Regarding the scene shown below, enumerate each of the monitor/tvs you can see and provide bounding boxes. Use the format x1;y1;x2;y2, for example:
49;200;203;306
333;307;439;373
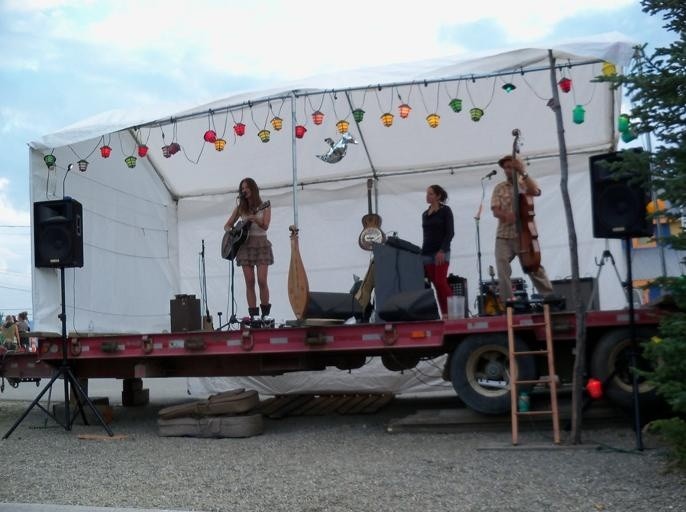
33;198;84;268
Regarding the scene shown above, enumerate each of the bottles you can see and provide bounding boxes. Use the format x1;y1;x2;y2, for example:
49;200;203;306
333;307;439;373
88;320;95;337
518;390;530;414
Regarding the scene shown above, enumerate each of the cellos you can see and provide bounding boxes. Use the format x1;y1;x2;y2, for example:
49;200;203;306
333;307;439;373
512;129;541;275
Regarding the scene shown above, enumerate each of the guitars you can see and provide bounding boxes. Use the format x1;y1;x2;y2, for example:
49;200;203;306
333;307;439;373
359;179;386;251
221;200;270;261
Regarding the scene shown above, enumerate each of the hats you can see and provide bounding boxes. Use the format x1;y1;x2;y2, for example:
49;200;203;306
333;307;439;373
498;154;521;164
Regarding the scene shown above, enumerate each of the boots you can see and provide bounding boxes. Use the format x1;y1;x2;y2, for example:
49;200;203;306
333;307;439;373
260;304;274;322
248;308;261;323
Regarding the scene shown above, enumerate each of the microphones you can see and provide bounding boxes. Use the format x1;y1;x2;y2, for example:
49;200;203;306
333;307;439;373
200;239;205;258
486;169;497;178
237;191;246;199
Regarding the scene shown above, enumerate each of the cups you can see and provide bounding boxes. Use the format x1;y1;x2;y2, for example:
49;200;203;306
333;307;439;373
446;296;465;320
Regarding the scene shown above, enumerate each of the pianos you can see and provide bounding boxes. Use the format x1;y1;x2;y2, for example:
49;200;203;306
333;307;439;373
387;237;422;254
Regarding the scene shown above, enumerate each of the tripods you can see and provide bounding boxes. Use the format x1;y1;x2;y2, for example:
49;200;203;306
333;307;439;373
615;240;672;454
213;199;245;331
2;269;114;440
585;239;628;310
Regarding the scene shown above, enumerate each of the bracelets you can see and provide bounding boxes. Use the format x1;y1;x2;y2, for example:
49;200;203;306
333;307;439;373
522;173;528;180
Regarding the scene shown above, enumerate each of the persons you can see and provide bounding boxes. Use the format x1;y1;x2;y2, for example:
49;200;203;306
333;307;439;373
422;184;455;321
491;156;556;303
224;177;274;320
0;312;31;351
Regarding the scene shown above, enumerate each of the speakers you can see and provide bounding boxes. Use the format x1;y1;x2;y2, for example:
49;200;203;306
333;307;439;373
170;298;201;332
588;148;655;240
302;291;372;324
376;289;441;320
